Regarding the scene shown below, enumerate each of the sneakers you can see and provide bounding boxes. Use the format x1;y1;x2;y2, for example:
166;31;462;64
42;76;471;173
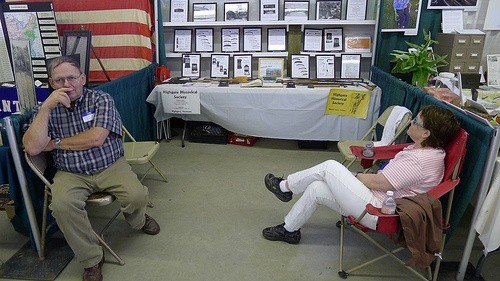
83;248;104;281
263;222;302;244
142;213;160;235
265;173;293;202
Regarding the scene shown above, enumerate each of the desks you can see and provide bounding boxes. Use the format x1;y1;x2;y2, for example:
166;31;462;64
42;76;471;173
146;76;382;148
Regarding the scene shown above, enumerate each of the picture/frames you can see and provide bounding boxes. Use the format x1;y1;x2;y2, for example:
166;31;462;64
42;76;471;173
426;0;479;10
381;0;423;32
62;29;93;86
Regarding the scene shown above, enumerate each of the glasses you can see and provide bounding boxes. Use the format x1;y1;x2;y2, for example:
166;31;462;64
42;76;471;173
413;117;423;127
52;73;82;85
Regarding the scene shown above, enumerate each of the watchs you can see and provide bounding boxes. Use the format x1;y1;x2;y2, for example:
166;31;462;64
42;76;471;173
352;171;362;177
55;137;63;150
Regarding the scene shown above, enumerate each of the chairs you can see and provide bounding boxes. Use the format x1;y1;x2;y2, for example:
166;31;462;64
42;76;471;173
121;122;167;208
337;126;469;281
25;144;125;266
338;105;412;174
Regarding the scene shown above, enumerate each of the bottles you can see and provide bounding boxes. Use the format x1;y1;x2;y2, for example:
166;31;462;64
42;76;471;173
362;141;375;158
380;190;396;215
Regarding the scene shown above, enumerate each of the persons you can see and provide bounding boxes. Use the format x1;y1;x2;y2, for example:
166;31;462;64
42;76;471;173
22;56;160;281
263;105;461;244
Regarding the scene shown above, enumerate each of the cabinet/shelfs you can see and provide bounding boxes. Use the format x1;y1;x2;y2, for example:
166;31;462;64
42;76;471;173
434;31;486;74
162;17;376;58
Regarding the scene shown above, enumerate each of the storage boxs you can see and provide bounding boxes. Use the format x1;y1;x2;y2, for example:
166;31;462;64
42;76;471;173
187;120;228;144
